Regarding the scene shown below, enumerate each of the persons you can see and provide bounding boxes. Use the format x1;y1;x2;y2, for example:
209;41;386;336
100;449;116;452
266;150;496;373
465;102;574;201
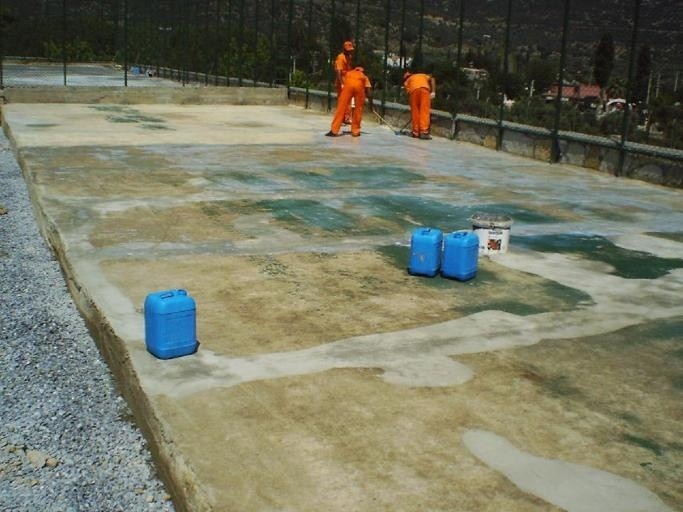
334;40;354;126
324;66;374;137
402;70;435;139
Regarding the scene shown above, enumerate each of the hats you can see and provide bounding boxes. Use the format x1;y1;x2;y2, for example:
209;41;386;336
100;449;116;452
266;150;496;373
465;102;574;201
343;40;355;52
403;73;412;79
355;66;364;71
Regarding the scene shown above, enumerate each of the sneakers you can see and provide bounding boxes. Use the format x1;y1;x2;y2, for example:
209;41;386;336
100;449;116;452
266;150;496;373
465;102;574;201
419;133;433;139
325;130;336;136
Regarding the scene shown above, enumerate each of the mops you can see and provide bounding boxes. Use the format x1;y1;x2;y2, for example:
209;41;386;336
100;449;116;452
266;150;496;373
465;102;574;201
372;109;413;136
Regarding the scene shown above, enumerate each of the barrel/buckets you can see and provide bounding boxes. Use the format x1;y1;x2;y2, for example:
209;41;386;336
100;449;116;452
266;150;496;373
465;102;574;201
407;227;444;278
441;231;480;281
467;211;514;256
142;289;200;360
132;66;140;75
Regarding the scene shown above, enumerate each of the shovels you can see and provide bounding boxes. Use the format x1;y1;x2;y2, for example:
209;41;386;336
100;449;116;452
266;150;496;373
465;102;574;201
373;111;405;136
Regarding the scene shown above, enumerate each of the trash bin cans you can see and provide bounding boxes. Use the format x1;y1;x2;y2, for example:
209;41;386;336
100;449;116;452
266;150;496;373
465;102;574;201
471;213;514;253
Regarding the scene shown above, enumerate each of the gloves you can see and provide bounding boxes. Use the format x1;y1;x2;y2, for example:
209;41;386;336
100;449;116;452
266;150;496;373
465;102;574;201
430;92;435;99
